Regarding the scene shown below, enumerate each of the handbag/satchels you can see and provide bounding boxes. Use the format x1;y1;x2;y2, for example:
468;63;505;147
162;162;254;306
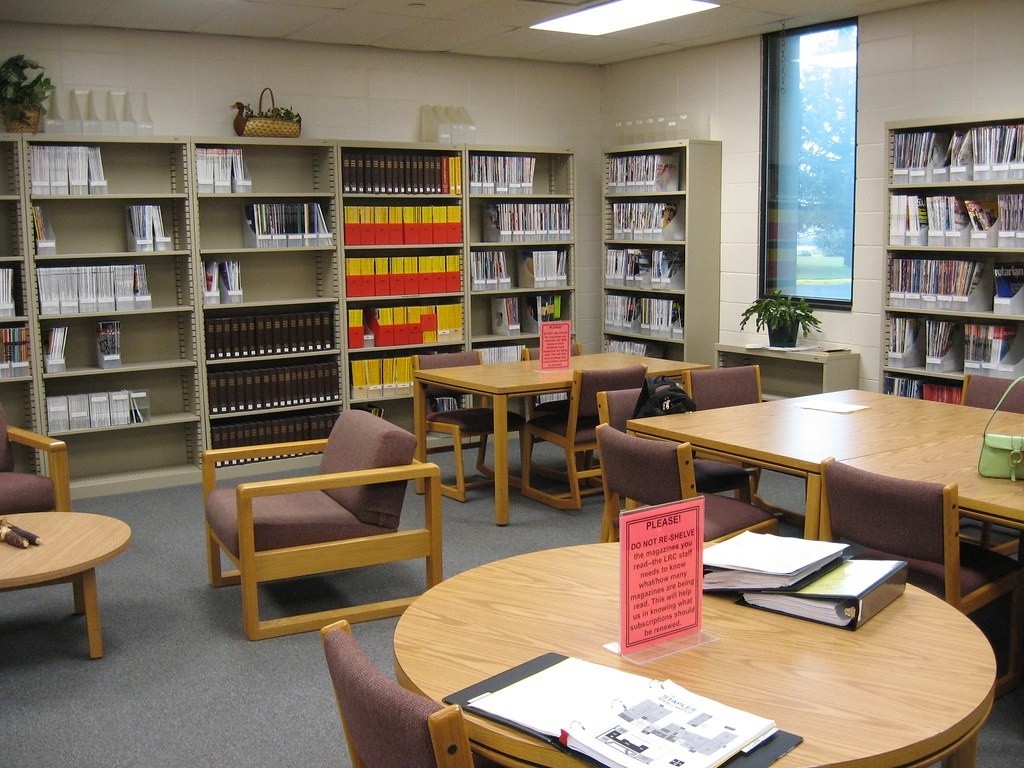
977;376;1024;481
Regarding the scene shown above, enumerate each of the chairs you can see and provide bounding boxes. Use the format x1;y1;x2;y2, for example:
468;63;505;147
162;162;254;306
207;409;445;640
522;342;584;359
413;352;527;501
321;620;473;767
959;374;1024;415
681;366;765;409
1;426;76;513
596;423;779;547
596;385;755;504
818;456;1024;700
519;367;645;510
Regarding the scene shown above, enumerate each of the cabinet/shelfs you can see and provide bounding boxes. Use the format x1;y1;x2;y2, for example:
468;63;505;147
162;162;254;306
192;133;344;467
338;139;468;449
880;116;1024;408
22;134;205;500
1;132;45;480
596;139;721;368
713;343;860;402
466;145;575;362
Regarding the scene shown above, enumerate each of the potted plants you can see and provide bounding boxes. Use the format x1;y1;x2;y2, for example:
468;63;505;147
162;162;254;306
742;292;819;349
1;55;53;134
231;88;301;138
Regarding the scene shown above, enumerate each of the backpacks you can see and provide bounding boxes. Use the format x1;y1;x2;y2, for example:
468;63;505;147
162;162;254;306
633;375;696;459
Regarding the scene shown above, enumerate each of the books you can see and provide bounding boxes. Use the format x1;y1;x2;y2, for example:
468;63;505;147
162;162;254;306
605;153;682;358
0;269;15;316
887;123;1024;407
201;260;242;305
245;202;332;247
469;156;570;404
47;387;144;432
32;205;47;255
36;263;149;315
195;146;245;193
0;327;29;377
341;149;463;418
128;204;166;251
31;146;106;195
42;325;68;373
204;312;341;466
96;321;121;367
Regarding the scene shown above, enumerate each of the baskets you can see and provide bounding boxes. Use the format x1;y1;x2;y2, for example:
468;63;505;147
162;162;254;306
2;103;40;133
240;87;301;137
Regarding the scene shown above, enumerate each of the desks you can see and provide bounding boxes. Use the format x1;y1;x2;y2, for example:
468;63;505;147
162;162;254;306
410;353;712;526
393;539;996;768
627;389;1024;545
1;507;130;661
